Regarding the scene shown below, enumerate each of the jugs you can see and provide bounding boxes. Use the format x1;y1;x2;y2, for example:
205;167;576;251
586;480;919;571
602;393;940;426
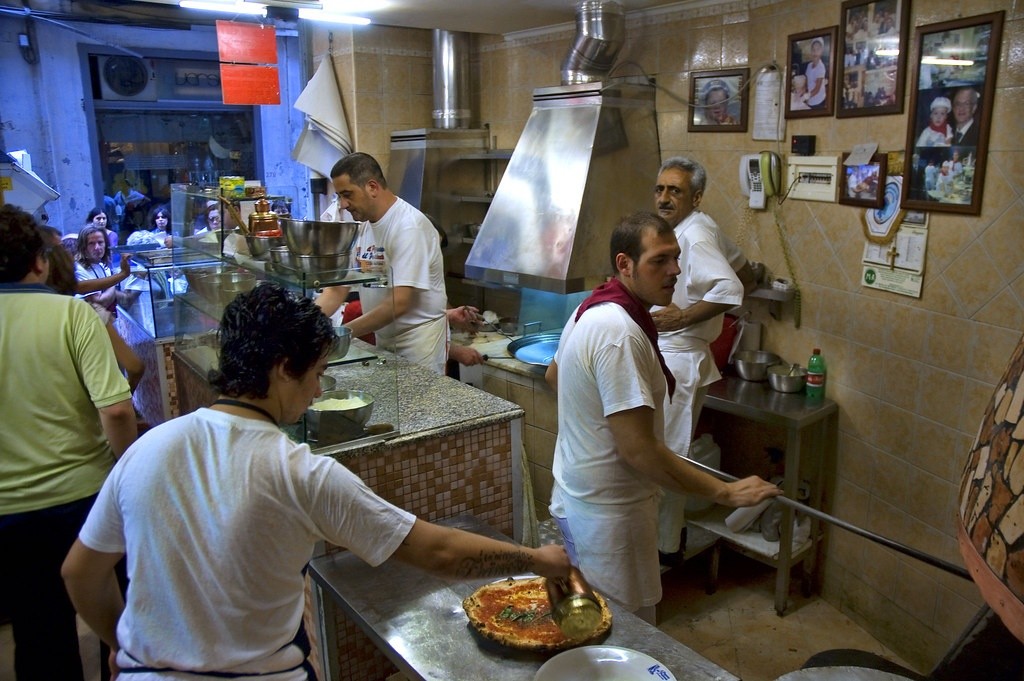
217;195;282;236
545;565;602;637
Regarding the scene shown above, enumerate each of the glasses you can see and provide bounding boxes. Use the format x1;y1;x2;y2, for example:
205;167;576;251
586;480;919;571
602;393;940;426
207;216;222;222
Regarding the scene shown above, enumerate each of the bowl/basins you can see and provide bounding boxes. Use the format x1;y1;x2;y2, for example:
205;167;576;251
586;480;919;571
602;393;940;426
304;374;375;438
183;266;279;310
496;315;517;330
248;217;361;281
733;351;782;381
468;223;482;238
475;319;497;332
766;365;808;393
330;325;352;361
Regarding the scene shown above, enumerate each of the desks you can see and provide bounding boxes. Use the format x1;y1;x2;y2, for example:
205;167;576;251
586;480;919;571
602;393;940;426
686;372;837;617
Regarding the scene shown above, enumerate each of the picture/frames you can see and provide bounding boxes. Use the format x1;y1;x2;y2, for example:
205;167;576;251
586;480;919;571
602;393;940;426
836;0;911;120
686;67;749;133
784;24;839;120
899;10;1007;216
839;151;888;210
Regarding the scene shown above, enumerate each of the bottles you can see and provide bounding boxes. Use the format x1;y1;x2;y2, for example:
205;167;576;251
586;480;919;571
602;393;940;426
806;347;825;400
682;435;723;519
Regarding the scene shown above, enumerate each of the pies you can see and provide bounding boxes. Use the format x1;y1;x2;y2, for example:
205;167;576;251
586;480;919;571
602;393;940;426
462;575;613;650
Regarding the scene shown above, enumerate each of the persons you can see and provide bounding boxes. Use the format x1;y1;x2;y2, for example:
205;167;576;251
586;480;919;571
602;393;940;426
794;37;826;109
164;199;252;273
115;230;164;334
951;88;980;146
39;207;144;394
916;98;953;146
650;157;757;554
699;79;736;125
313;152;451;376
151;208;172;236
104;179;150;231
0;201;136;681
919;34;962;90
271;200;291;214
546;212;783;626
340;300;485;367
61;281;571;681
908;150;962;202
847;167;877;198
843;9;896;107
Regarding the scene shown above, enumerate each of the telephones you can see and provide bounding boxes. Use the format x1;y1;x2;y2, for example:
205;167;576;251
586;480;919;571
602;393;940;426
739;154;767;209
761;151;781;196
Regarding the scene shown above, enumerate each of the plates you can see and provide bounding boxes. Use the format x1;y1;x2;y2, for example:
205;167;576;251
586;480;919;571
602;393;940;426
293;420;366;442
533;645;677;681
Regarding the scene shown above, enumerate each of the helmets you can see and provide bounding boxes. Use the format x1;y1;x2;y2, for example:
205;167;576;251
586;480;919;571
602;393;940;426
930;97;952;113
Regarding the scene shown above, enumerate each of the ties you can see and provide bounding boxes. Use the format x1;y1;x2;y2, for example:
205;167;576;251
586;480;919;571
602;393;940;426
929;119;947;137
955;131;963;142
574;277;675;403
